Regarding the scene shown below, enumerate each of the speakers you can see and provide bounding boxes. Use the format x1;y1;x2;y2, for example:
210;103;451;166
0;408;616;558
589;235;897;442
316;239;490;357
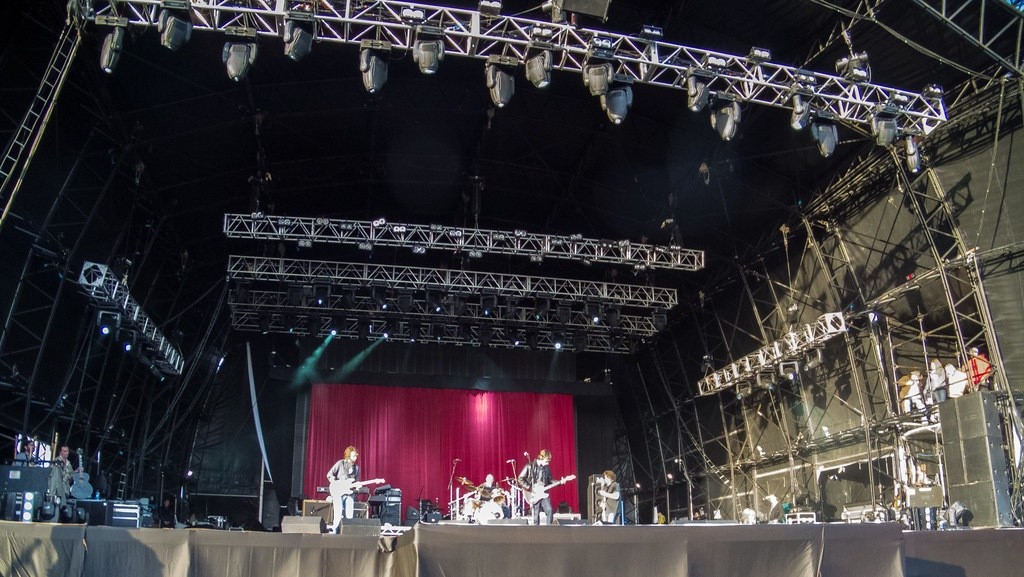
487;519;528;524
587;483;603;518
551;518;591;525
281;499;443;536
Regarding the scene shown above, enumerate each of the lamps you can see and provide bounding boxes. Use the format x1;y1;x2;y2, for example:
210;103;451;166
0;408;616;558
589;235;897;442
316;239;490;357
222;26;259;83
522;42;554;89
600;74;635;124
710;92;743;141
681;67;713;112
284;10;316;63
703;56;726;71
485;55;520;109
583;51;613;96
528;26;553;40
589;36;613;50
156;1;193;50
412;24;446;76
400;6;425;24
749;46;943;179
94;215;823;403
478;0;504;15
95;14;128;72
639;25;664;40
358;37;391;94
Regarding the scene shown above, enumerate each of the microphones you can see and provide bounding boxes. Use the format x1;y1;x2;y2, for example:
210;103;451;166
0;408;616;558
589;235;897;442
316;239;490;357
454;459;460;461
311;509;313;516
524;452;529;456
506;459;515;463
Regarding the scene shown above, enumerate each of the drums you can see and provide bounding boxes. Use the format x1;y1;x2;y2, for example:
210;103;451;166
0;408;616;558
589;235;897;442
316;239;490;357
491;488;505;504
463;498;481;516
474;502;504;524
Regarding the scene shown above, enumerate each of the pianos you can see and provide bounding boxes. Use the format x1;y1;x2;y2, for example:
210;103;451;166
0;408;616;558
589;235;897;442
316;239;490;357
316;486;370;525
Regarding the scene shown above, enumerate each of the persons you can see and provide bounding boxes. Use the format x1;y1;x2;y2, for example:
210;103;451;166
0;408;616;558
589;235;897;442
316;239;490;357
923;358;946;423
54;445;84;495
944;363;967;399
598;470;620;524
960;347;993;393
694;509;704;520
896;369;928;425
475;474;499;499
16;440;36;467
326;446;362;534
517;449;566;525
763;494;783;522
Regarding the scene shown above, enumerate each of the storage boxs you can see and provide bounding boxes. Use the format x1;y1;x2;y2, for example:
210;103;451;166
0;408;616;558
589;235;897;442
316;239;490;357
112;504;143;528
786;512;816;525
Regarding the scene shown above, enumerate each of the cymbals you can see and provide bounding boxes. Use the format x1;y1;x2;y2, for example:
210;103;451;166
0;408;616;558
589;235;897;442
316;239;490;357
456;477;473;484
466;486;488;495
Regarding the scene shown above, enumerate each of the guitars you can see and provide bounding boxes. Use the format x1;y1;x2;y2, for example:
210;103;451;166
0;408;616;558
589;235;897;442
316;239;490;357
69;448;94;499
329;478;385;499
523;474;577;505
601;483;606;512
